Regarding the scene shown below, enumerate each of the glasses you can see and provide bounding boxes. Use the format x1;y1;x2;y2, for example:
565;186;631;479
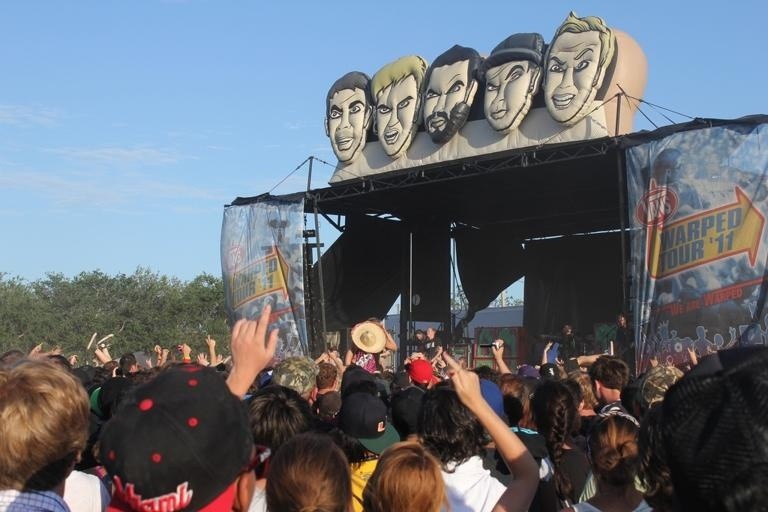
237;445;271;479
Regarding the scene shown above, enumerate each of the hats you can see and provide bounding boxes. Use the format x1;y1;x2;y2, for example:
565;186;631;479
479;379;504;417
88;371;256;512
273;356;320;393
539;363;560;382
351;321;386;354
342;360;438;455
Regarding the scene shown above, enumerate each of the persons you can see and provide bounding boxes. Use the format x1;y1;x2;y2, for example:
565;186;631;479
484;32;545;134
542;9;617;126
423;44;483;147
323;69;373;165
632;127;768;338
221;202;308;360
367;55;428;159
